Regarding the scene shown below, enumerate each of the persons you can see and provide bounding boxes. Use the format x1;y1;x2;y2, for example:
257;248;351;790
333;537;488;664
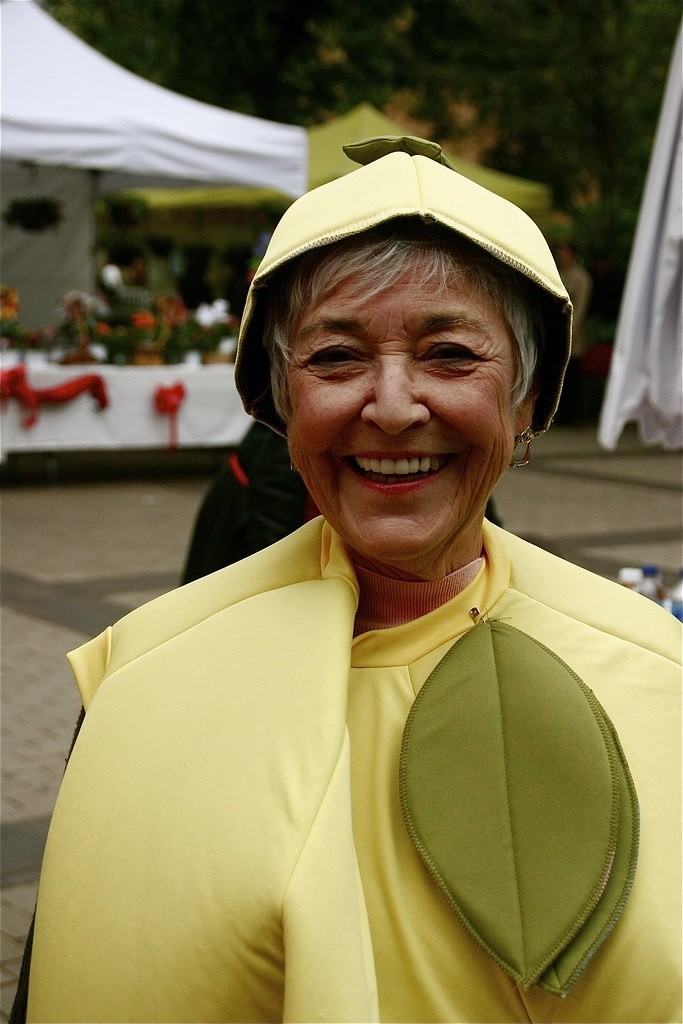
11;136;683;1022
0;285;95;352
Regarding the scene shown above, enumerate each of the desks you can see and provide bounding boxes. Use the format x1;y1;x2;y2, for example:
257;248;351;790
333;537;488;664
2;343;254;483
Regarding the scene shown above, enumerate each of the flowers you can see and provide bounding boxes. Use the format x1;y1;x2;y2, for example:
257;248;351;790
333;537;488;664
0;269;238;366
154;384;184;414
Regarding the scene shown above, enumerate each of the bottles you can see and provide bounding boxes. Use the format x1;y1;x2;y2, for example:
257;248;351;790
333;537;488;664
640;567;667;607
668;566;683;624
617;566;644;591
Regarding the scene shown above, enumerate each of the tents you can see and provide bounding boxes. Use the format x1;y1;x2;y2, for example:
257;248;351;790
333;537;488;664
2;0;548;233
